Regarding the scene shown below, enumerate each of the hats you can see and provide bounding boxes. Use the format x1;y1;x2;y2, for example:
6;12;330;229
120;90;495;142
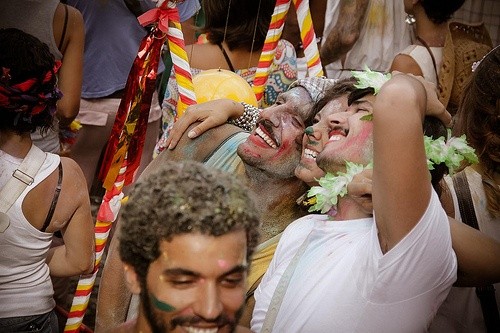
437;20;495;110
288;78;340;103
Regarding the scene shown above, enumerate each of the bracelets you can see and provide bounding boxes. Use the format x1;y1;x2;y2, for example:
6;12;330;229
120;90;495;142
229;101;263;132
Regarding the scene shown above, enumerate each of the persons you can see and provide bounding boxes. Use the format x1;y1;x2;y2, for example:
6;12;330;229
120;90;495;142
0;0;500;333
